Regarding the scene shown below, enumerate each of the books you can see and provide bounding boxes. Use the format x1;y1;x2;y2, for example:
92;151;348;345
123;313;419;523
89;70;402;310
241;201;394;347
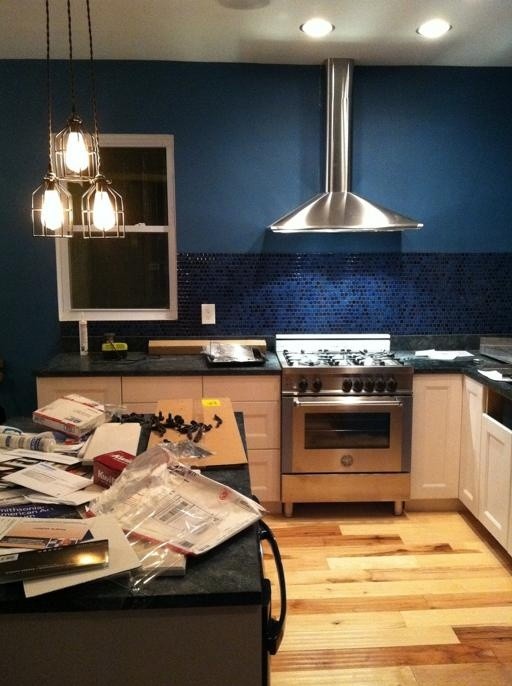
81;421;143;466
33;397;106;439
104;540;187;579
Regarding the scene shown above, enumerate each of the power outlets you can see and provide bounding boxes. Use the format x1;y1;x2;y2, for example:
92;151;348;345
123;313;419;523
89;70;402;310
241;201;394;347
202;304;216;324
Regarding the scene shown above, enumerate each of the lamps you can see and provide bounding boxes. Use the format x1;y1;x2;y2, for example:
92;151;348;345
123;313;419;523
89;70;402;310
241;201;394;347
32;0;75;238
81;0;126;240
54;0;98;182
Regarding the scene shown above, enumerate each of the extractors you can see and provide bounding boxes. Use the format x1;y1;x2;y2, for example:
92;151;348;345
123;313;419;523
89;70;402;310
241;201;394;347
266;58;424;234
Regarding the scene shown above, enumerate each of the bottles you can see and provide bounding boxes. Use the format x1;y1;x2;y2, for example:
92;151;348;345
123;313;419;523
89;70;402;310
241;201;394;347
0;434;71;453
79;312;88;356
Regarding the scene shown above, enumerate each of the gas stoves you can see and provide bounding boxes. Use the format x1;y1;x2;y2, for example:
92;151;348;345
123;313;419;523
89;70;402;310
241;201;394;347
277;349;414;395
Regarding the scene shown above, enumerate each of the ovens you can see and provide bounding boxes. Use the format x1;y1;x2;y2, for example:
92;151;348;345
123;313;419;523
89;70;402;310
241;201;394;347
281;395;413;517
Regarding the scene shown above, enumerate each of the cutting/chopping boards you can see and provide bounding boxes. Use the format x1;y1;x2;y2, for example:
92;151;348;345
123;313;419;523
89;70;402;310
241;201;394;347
81;469;201;577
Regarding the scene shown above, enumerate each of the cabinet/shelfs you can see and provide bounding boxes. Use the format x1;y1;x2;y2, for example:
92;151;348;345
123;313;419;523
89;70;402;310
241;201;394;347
411;368;463;512
458;369;487;531
37;370;122;421
122;370;203;414
203;369;282;514
50;134;179;322
479;377;512;557
